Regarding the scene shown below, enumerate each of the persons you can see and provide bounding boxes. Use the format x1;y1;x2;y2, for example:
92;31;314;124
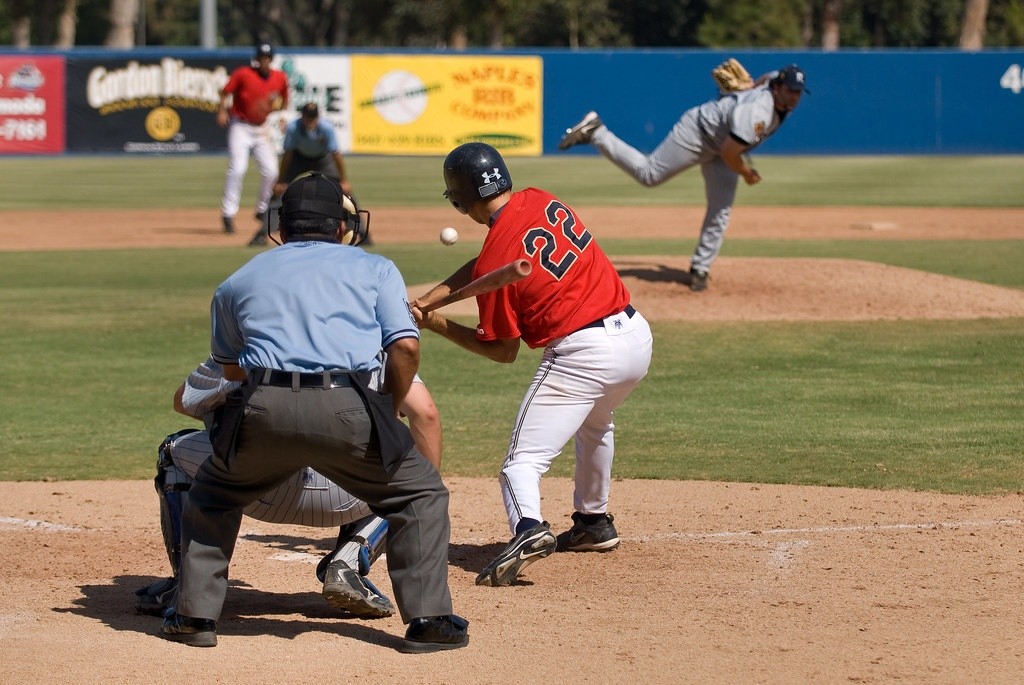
248;102;373;245
140;171;469;653
410;142;653;583
216;43;288;234
558;58;810;292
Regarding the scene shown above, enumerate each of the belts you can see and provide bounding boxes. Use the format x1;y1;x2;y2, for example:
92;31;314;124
568;303;637;335
698;110;707;136
258;369;354;388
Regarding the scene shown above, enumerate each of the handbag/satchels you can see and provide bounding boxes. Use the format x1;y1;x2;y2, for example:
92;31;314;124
351;371;415;474
210;366;265;465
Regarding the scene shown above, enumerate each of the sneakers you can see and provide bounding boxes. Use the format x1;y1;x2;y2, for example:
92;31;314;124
560;110;603;149
400;614;470;653
690;268;711;292
158;606;218;646
555;512;621;554
475;520;557;588
135;576;177;618
322;560;396;619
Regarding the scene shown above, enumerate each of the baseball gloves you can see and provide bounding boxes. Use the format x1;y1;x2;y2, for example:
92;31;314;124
712;57;755;93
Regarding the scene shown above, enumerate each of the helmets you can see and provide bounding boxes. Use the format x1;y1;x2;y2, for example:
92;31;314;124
443;142;512;215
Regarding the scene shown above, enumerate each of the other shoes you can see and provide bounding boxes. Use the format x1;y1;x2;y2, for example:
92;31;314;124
256;211;266;221
222;215;236;233
249;230;266;247
359;230;373;245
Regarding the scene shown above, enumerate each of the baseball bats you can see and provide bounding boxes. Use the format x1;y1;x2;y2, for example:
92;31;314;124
419;259;532;312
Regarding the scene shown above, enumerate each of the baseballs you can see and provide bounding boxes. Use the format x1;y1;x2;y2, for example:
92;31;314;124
439;227;459;246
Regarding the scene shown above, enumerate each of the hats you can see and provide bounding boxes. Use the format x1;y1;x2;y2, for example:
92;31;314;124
301;104;318;118
281;172;343;220
256;44;272;63
779;66;811;95
